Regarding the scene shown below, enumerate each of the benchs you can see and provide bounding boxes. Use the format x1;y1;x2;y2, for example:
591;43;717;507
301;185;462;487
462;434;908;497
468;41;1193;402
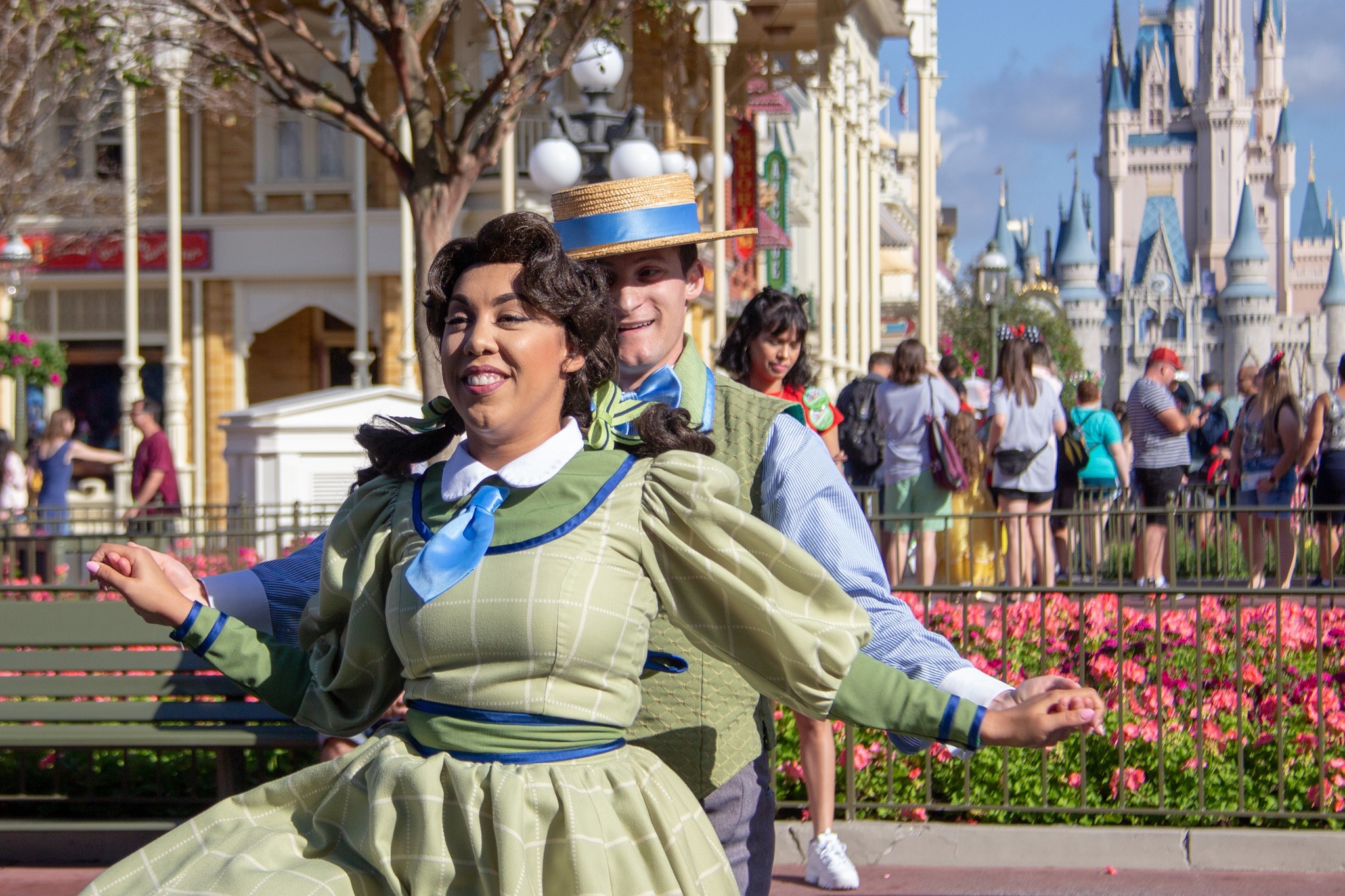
0;601;332;832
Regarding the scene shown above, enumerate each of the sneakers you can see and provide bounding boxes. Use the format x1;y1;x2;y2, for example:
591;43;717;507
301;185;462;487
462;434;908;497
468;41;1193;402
804;828;859;890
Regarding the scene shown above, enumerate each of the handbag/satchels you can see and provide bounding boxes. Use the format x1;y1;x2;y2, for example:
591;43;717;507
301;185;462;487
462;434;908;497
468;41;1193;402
1199;429;1235;497
997;449;1037;474
923;377;967;494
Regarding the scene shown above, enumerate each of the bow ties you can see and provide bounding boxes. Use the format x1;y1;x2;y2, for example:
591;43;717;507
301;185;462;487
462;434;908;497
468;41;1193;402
590;363;682;410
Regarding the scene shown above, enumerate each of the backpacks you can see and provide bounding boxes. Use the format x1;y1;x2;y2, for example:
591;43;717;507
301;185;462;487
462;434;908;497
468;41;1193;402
1073;408;1103;453
1193;397;1231;456
838;377;884;471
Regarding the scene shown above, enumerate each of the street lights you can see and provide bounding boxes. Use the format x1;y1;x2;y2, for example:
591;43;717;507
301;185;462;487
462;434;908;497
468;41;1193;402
93;3;198;560
974;242;1011;382
0;232;36;463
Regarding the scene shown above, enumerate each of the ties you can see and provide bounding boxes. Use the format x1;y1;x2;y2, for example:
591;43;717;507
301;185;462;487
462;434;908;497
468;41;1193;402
404;486;512;602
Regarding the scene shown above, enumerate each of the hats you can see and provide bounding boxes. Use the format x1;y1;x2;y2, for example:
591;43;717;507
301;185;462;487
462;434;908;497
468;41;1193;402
1201;371;1225;386
550;172;757;262
1146;348;1182;371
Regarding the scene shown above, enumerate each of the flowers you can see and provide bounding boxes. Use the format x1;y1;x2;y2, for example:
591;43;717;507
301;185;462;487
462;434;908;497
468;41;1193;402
0;331;69;389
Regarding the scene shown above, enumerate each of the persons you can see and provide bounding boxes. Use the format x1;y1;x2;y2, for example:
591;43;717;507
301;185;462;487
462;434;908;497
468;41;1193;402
78;213;1106;896
835;351;894;531
122;399;182;556
875;339;961;611
108;172;1107;896
938;354;966;401
1182;371;1242;540
934;410;1006;604
1125;348;1210;600
717;287;859;889
1068;381;1133;571
25;408;127;537
1299;354;1345;588
984;337;1077;604
0;430;29;535
1229;351;1302;591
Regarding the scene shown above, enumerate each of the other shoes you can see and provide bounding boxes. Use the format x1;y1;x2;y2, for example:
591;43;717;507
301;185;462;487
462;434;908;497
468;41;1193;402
1055;573;1068;584
1310;571;1335;586
1139;576;1184;600
